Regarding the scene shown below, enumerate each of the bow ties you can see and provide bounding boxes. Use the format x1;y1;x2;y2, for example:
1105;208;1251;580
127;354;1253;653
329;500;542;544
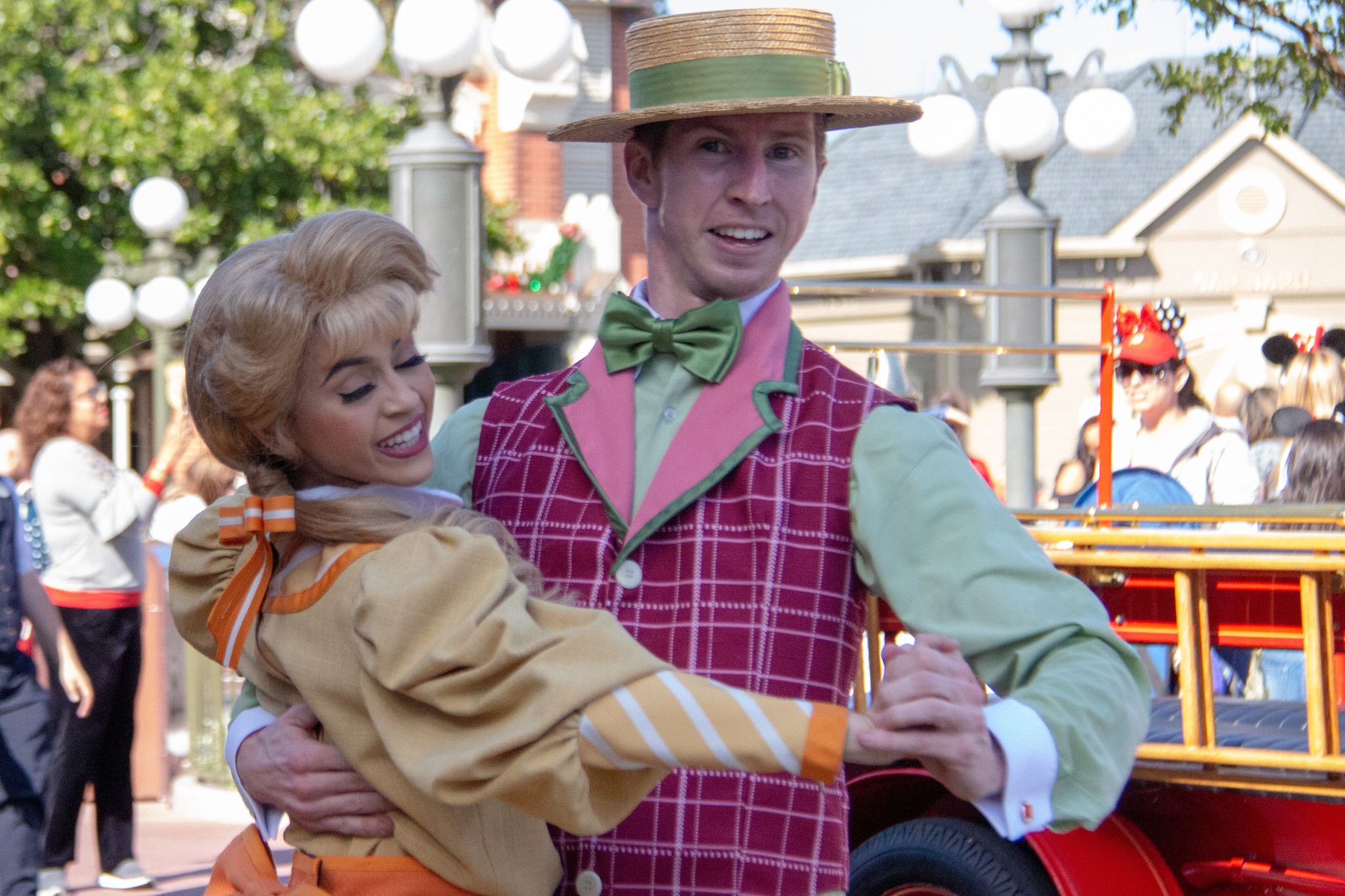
596;289;742;384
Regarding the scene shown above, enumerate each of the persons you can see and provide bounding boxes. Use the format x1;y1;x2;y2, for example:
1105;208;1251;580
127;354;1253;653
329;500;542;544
1093;299;1259;700
1054;414;1099;528
1216;329;1345;499
14;356;192;896
1258;419;1345;703
225;8;1151;896
170;210;953;896
903;393;992;492
0;472;93;896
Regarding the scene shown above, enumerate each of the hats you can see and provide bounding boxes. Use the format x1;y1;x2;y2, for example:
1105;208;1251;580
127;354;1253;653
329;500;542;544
544;7;922;143
1113;298;1184;366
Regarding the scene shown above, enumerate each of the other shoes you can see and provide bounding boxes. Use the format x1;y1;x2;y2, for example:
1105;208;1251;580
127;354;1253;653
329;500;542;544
36;867;68;896
97;860;154;889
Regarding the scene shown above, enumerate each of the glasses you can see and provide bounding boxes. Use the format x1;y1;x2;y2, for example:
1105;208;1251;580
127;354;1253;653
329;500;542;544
73;382;107;404
1115;362;1171;383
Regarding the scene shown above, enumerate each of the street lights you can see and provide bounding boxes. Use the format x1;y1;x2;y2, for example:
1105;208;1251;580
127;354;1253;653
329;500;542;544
910;8;1139;527
295;0;574;442
82;175;210;720
